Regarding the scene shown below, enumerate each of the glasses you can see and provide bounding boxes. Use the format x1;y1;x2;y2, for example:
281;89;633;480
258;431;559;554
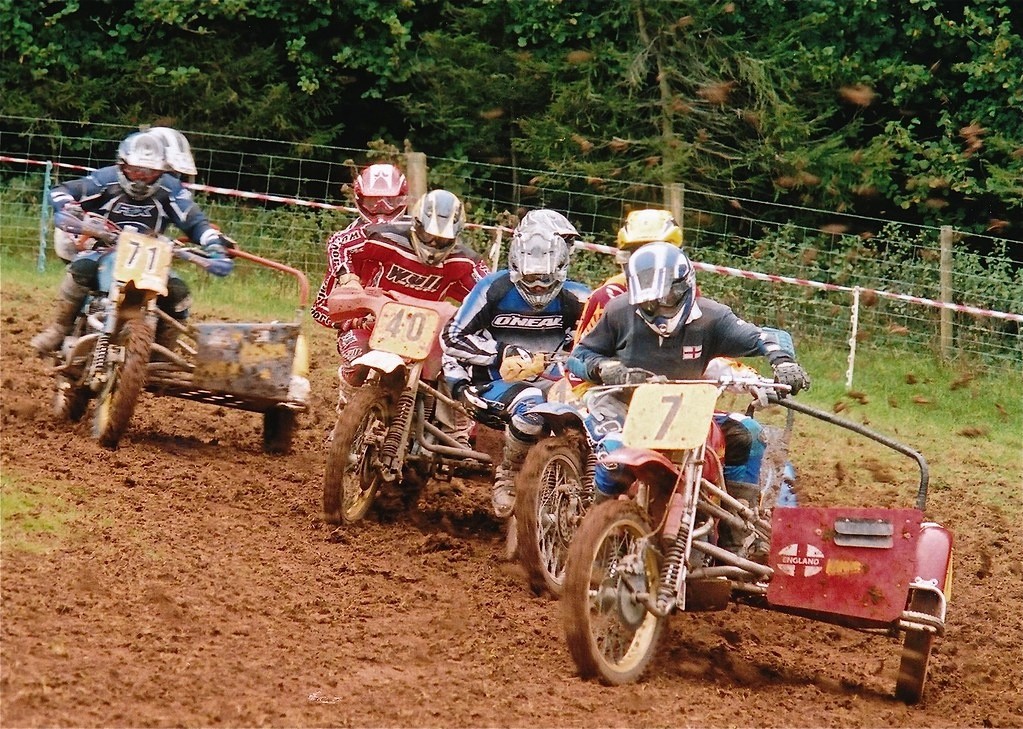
114;154;164;185
412;216;458;253
354;181;409;218
508;263;570;288
638;266;696;319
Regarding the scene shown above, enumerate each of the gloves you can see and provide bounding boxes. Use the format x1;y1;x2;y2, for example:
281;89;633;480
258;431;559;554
202;243;231;277
495;340;535;365
769;361;812;400
581;389;629;423
65;206;86;222
460;383;506;430
596;360;628;385
333;272;368;332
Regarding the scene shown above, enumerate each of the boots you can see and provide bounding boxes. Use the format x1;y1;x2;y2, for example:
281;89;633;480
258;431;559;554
328;364;363;442
589;478;626;569
442;408;476;461
150;315;190;388
28;273;92;355
715;481;768;583
491;424;539;520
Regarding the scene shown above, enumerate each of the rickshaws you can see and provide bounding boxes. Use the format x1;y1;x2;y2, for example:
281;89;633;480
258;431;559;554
324;285;506;530
560;365;960;705
507;342;796;606
52;209;312;455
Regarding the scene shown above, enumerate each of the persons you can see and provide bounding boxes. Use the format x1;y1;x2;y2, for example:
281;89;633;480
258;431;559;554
438;209;594;517
567;210;810;585
311;165;492;464
30;127;234;362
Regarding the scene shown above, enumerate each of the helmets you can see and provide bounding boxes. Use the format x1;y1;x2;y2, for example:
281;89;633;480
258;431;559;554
143;126;199;194
613;209;684;251
113;132;168;201
626;241;697;340
410;189;468;268
352;164;410;226
507;210;582;313
